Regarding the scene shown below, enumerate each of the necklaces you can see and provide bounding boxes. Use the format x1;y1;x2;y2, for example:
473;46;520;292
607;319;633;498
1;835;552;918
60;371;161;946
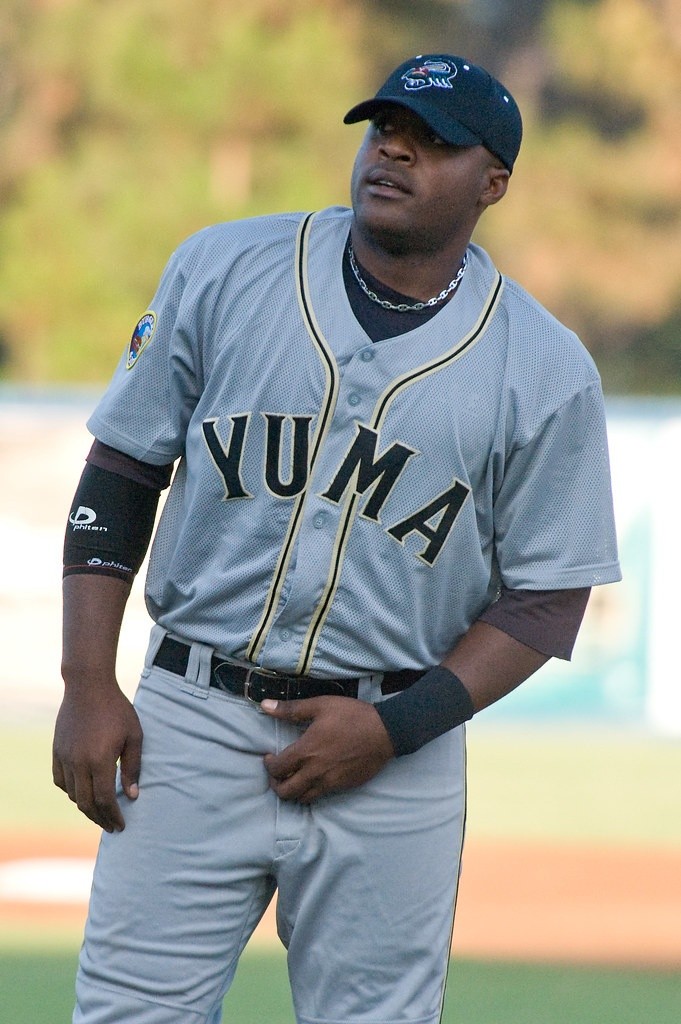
348;239;467;310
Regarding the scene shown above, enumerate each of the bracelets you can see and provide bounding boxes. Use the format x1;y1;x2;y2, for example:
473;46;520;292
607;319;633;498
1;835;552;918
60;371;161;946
373;664;474;757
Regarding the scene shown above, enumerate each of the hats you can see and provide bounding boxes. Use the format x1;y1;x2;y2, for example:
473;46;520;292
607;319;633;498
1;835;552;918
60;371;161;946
343;54;523;177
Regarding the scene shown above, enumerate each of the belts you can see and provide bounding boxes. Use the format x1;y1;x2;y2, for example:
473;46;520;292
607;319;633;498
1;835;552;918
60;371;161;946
152;636;429;709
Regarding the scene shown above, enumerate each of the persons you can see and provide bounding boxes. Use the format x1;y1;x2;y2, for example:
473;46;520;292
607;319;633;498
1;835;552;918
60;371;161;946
53;52;622;1023
